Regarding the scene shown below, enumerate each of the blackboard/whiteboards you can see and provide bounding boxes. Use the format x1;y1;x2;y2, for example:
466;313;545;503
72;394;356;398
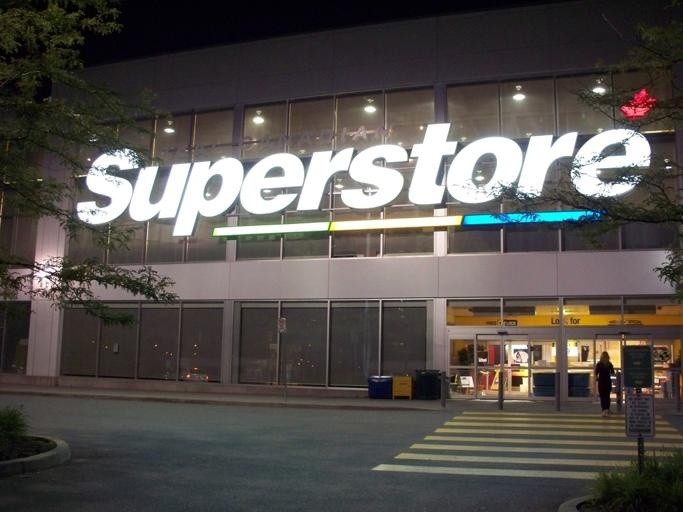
459;375;474;388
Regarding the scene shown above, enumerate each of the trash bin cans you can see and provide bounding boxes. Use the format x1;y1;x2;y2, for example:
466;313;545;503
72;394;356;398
414;370;440;399
368;376;393;398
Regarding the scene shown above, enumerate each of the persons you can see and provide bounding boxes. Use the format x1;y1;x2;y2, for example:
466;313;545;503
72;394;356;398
515;352;521;362
593;350;615;416
